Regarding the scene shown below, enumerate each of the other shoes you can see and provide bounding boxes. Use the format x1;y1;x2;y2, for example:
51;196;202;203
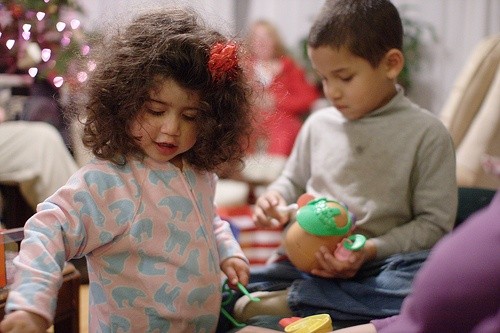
234;288;295;325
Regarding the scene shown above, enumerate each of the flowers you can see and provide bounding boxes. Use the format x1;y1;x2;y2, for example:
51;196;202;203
210;43;240;82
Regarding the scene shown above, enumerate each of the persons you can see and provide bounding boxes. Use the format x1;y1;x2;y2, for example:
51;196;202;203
242;19;318;203
222;0;457;322
0;72;80;210
0;0;295;333
234;189;500;333
